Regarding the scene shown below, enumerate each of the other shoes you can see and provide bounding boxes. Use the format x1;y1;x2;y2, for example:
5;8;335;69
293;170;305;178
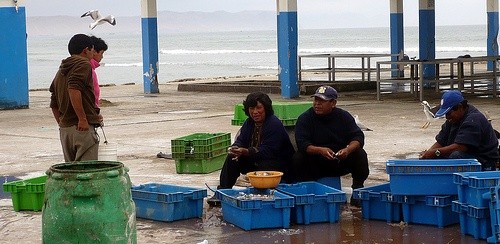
350;196;362;207
207;185;226;204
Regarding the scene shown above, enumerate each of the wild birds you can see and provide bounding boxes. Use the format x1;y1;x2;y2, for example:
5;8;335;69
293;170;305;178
353;112;373;132
79;10;117;30
421;100;442;129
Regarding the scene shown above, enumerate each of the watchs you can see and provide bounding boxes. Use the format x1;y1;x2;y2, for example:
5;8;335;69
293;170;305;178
434;149;441;157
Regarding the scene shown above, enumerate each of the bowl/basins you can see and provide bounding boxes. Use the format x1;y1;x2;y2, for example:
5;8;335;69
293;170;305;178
247;171;284;188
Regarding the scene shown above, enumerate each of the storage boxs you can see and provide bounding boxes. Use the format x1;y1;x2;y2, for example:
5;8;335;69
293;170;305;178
280;175;342;190
275;182;347;225
3;176;49;213
216;187;294;232
131;183;207;222
0;175;23;199
352;159;500;244
231;100;314;127
171;133;233;174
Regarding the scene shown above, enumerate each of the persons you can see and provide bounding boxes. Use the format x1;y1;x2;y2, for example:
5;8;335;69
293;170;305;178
88;35;108;109
48;34;103;161
294;85;369;208
418;90;500;171
207;91;297;204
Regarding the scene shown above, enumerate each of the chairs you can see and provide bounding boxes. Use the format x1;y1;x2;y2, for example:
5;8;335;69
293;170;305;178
421;101;442;129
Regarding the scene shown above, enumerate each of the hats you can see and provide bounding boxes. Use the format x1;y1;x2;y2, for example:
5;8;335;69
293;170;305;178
310;86;338;101
435;90;464;116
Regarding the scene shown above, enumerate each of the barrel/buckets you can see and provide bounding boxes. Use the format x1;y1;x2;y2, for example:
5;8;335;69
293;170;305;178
41;160;136;244
